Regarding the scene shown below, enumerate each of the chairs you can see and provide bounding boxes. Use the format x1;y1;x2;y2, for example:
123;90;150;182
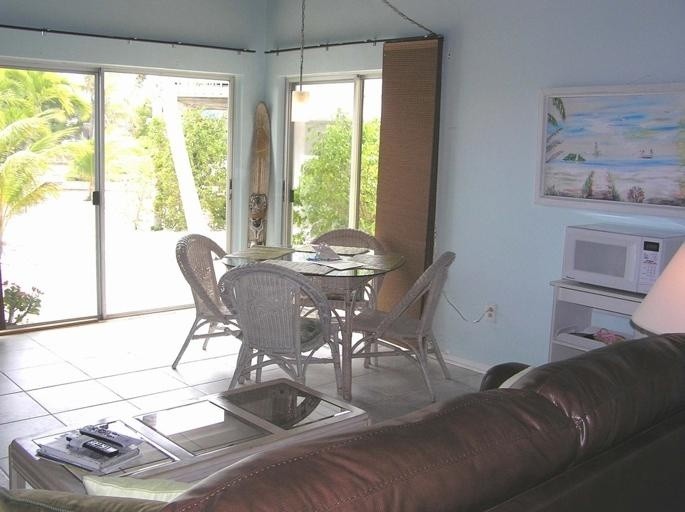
172;233;242;370
341;252;455;402
215;263;342;394
301;228;386;368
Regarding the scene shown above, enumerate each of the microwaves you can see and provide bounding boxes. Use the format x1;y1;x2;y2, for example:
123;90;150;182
559;220;685;295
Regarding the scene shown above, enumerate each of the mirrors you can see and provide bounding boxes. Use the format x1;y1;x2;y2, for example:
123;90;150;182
248;100;268;246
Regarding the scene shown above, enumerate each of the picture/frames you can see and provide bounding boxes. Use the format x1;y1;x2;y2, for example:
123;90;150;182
533;83;685;217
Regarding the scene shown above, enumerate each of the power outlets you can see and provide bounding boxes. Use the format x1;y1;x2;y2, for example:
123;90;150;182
483;304;496;322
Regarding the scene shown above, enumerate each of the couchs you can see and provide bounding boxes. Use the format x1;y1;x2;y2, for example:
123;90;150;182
2;332;684;510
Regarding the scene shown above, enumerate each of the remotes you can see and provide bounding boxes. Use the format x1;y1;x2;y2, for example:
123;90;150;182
80;425;131;447
66;432;119;456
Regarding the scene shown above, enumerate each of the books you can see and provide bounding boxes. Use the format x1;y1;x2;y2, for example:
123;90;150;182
35;429;145;472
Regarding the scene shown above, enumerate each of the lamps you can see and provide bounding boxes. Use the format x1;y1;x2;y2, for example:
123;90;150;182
291;1;311;105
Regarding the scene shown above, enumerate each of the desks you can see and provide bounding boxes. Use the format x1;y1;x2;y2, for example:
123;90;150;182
220;245;406;402
545;280;646;369
